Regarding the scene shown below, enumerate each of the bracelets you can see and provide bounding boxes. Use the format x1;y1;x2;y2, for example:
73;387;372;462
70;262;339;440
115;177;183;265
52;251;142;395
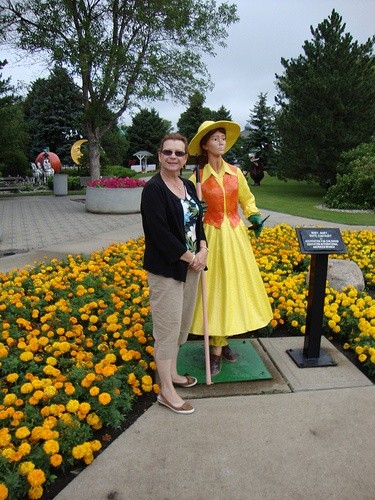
200;246;209;252
188;255;195;266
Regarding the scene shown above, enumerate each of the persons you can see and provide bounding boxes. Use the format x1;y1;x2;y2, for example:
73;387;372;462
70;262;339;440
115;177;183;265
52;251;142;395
189;168;196;186
187;121;274;375
140;134;209;414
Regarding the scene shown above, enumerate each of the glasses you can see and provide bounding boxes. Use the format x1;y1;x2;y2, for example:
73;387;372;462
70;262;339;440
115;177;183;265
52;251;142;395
161;150;186;157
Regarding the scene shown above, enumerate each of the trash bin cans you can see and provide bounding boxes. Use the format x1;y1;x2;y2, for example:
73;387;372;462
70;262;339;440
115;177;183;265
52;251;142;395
53;173;69;196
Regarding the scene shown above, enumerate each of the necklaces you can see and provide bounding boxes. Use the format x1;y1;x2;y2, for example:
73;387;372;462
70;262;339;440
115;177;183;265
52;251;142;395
166;179;181;190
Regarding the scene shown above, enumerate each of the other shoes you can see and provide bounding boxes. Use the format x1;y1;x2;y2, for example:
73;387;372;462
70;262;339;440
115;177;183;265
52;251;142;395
172;375;197;387
157;390;195;414
